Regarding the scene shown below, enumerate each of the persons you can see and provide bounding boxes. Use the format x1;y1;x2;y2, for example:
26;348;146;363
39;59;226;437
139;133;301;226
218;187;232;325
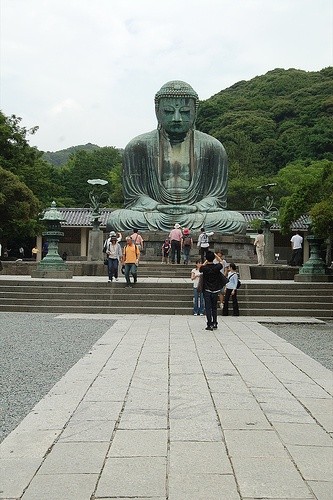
19;246;24;259
161;238;171;264
107;81;247;236
106;236;122;283
169;223;183;264
222;263;239;316
180;229;193;264
290;231;303;266
43;240;49;256
191;259;205;315
199;251;229;331
213;251;227;308
197;228;215;263
32;246;39;260
131;228;144;250
122;236;140;285
253;229;265;266
104;231;122;247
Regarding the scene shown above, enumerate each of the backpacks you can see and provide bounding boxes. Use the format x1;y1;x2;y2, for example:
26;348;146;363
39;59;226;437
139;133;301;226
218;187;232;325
201;233;208;243
184;236;190;244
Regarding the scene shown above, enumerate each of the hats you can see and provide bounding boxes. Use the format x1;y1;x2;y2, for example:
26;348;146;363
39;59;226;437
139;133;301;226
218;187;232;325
110;237;118;242
183;228;189;234
109;231;116;236
174;223;181;229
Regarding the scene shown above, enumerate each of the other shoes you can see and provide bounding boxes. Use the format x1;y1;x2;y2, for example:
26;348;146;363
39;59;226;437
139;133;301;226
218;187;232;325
172;262;174;263
200;313;203;316
125;285;132;288
205;325;213;331
194;313;197;316
108;280;112;283
220;303;223;309
115;278;119;281
257;264;261;266
213;324;217;329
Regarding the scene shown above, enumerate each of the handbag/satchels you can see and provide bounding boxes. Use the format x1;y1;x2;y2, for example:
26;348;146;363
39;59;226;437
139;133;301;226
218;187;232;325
236;279;241;290
198;267;206;293
102;241;107;253
107;244;110;258
